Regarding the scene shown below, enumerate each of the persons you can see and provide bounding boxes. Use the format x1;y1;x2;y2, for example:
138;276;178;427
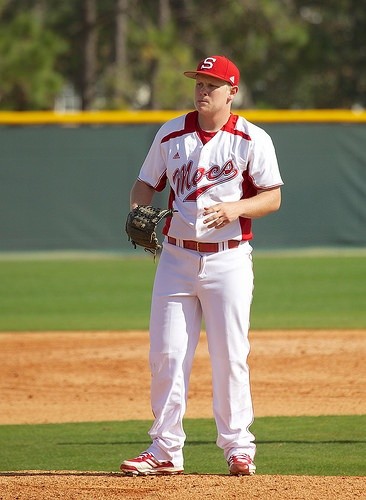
119;55;286;476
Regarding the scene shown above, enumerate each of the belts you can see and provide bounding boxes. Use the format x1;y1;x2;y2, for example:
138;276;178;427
168;236;240;253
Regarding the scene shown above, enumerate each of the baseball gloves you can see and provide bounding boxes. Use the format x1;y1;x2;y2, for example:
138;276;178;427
125;204;178;256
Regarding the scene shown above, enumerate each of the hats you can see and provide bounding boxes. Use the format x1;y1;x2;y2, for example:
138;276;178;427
183;55;240;87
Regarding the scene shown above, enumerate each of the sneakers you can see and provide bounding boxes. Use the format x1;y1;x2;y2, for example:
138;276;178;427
227;452;257;476
120;450;184;477
